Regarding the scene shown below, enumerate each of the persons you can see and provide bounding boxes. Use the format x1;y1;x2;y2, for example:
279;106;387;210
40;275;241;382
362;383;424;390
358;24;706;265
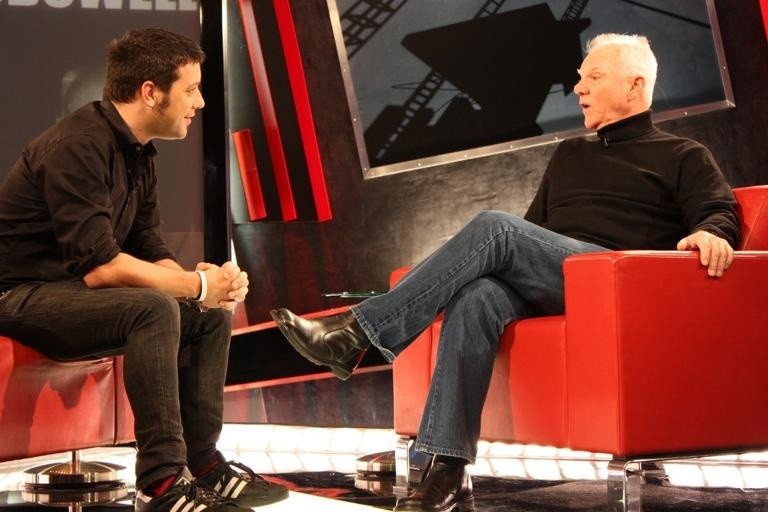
265;30;742;511
0;26;292;512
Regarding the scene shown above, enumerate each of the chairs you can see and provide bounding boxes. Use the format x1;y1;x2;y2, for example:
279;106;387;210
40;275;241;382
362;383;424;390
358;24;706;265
389;186;768;503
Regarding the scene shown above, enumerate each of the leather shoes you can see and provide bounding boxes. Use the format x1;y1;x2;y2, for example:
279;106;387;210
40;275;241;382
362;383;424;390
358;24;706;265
393;462;475;511
269;309;370;381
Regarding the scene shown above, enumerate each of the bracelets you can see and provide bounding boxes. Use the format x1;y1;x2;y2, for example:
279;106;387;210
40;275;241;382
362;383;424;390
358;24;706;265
192;270;208;303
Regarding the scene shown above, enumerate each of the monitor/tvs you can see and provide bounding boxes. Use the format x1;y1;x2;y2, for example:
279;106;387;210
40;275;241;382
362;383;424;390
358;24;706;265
0;0;231;271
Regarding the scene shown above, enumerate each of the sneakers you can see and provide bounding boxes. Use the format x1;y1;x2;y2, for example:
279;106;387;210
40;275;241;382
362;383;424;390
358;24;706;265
135;465;255;512
196;450;288;506
